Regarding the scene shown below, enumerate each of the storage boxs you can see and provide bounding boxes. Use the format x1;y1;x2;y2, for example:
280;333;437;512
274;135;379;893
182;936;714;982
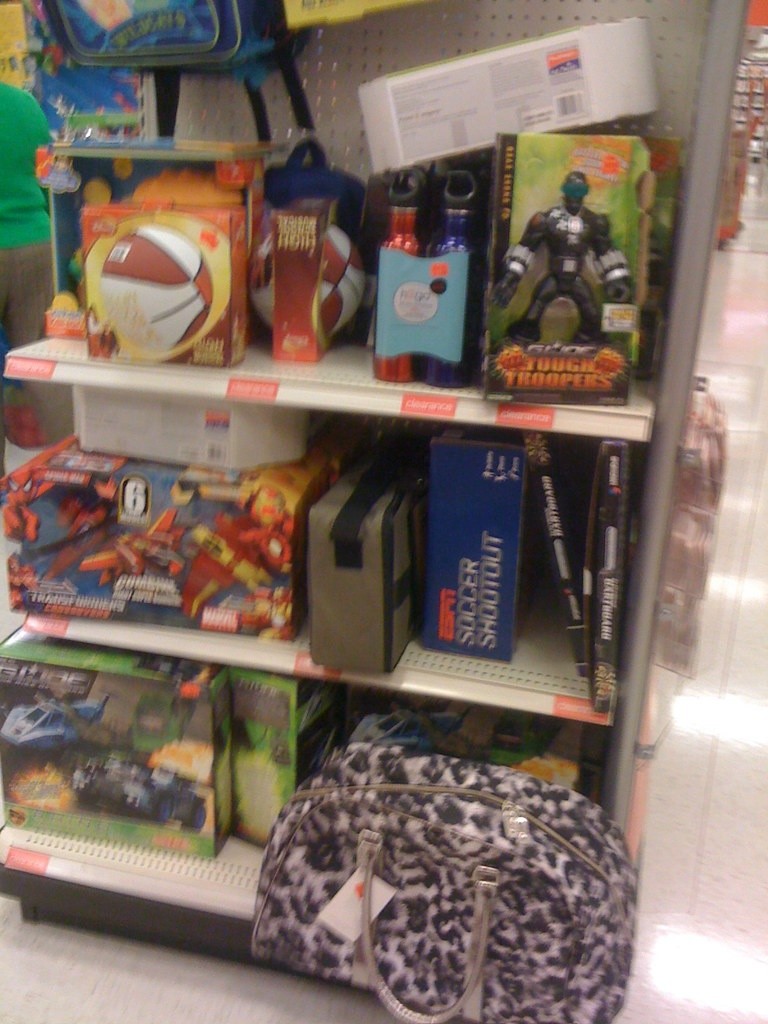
2;17;684;859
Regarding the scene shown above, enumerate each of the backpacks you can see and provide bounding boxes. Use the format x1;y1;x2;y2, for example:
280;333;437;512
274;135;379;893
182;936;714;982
42;0;284;71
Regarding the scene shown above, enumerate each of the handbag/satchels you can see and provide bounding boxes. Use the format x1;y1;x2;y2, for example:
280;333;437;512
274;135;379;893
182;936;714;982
250;742;639;1024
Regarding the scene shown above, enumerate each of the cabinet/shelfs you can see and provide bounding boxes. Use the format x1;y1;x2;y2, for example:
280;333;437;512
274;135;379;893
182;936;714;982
3;1;751;974
